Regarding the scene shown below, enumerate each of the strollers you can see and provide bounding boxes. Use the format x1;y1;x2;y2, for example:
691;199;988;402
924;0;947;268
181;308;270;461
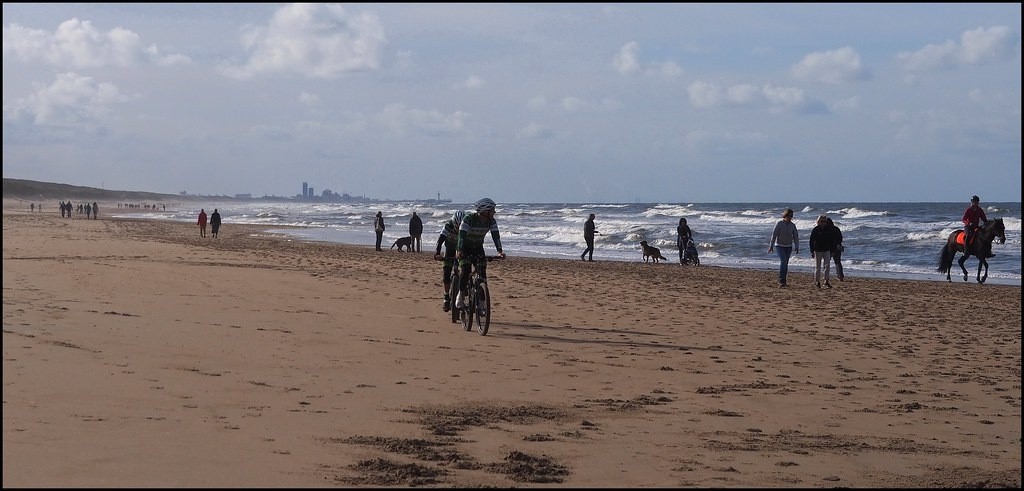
679;239;701;267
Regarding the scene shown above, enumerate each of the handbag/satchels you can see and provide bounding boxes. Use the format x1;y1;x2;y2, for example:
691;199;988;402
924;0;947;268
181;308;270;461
374;218;383;233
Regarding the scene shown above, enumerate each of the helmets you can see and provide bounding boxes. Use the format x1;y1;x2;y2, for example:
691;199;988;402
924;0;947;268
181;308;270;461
474;198;496;214
451;209;466;226
970;195;981;203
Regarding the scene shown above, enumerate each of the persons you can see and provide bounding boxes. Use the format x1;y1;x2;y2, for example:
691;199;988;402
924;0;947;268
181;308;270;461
676;217;698;265
962;194;997;258
58;200;74;217
76;202;99;220
374;210;385;252
766;209;800;287
197;208;207;238
580;214;599;262
809;213;845;289
408;212;424;253
210;209;222;238
30;203;34;212
117;202;166;211
434;197;506;316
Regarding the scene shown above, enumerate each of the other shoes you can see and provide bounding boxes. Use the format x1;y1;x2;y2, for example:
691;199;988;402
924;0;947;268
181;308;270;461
814;282;821;289
589;259;594;262
442;297;451;312
987;253;996;258
823;282;832;289
478;303;487;317
454;293;465;310
779;283;790;289
581;255;585;262
963;252;969;257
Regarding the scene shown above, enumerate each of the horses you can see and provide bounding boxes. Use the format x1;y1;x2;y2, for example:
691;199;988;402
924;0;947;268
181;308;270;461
937;218;1006;285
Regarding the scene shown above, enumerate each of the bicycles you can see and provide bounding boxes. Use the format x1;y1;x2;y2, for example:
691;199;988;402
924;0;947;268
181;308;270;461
456;254;504;337
434;255;464;323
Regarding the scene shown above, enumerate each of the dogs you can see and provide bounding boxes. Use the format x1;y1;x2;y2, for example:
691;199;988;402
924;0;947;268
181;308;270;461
391;237;411;252
639;241;667;263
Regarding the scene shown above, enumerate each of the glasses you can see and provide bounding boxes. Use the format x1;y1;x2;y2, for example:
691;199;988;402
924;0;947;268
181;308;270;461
818;219;827;222
